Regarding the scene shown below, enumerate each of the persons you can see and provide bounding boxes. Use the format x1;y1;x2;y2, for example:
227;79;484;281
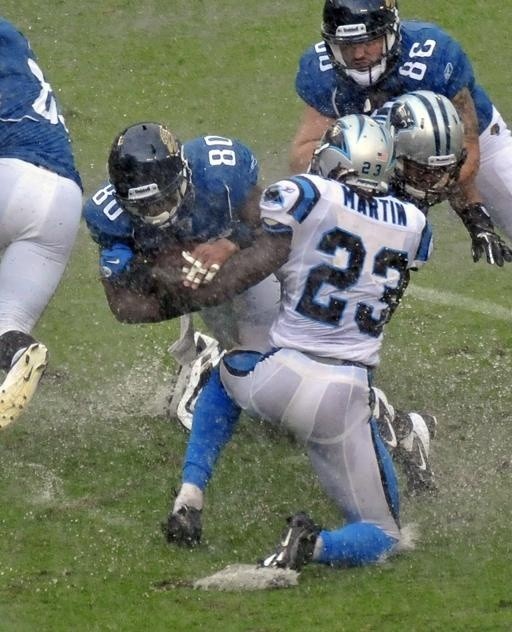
1;13;86;435
285;0;511;250
82;90;470;574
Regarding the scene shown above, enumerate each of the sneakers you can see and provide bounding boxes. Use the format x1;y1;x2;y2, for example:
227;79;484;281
393;412;440;497
257;511;323;574
2;342;51;428
177;349;227;431
162;503;203;549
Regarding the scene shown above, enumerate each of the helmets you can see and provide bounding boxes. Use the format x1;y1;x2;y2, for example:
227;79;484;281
307;113;398;197
321;1;405;44
384;90;469;170
108;121;185;198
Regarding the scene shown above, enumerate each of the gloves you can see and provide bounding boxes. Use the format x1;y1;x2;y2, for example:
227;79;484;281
461;203;512;267
